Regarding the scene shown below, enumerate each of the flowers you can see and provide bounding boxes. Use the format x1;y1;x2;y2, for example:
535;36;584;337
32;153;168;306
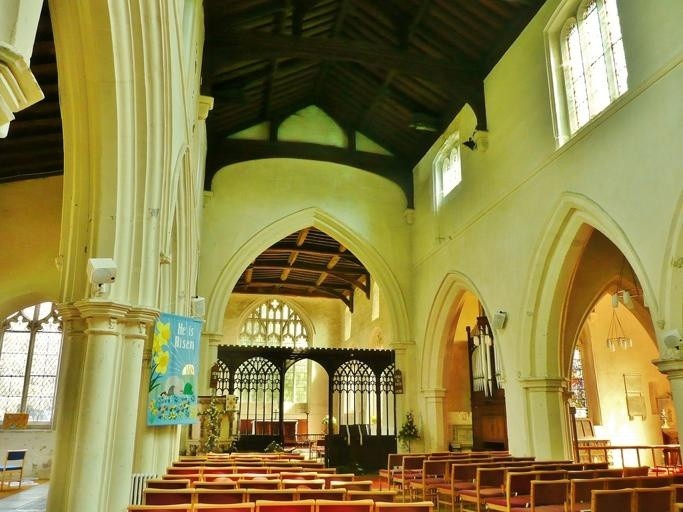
395;410;420;441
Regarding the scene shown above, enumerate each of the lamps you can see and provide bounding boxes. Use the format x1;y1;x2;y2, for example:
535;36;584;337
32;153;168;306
471;306;493;346
601;257;643;351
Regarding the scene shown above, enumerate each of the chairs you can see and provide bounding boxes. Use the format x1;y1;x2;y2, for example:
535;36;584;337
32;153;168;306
0;449;26;490
